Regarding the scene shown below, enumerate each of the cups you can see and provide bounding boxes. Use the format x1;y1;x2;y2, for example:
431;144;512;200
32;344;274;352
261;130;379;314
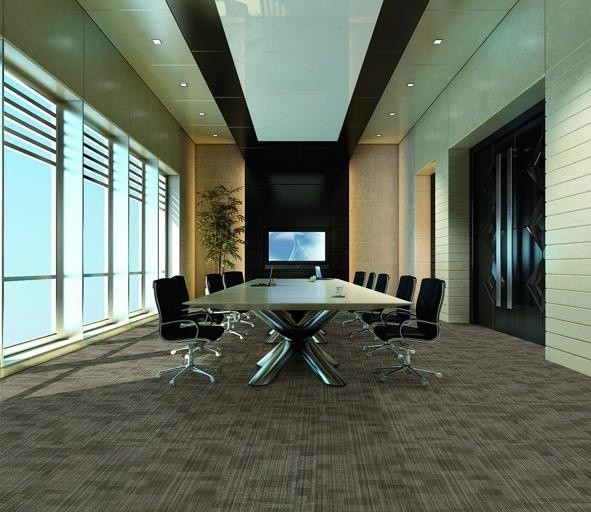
310;277;314;281
314;275;317;279
336;287;344;296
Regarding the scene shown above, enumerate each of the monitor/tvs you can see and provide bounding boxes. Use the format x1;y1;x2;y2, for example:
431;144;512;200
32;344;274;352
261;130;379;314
264;226;330;264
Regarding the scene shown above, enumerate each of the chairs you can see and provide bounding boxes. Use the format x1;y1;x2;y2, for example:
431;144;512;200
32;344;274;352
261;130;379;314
152;270;447;388
